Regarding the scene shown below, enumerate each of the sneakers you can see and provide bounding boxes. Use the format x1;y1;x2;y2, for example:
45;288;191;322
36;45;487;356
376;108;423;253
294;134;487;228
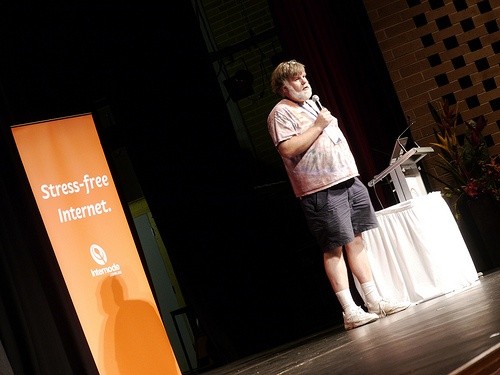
342;311;379;330
367;300;411;316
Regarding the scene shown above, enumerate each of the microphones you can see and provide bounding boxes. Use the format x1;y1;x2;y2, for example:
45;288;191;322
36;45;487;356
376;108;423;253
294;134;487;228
312;94;323;110
397;120;415;153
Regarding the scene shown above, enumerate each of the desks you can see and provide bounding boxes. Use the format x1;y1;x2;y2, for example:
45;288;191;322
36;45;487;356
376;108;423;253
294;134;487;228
350;190;479;317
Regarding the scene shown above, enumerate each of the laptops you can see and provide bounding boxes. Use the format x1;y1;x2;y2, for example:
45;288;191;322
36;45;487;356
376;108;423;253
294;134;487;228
388;137;408;165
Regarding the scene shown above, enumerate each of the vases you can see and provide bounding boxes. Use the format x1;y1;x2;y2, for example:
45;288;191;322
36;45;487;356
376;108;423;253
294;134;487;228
459;193;500;271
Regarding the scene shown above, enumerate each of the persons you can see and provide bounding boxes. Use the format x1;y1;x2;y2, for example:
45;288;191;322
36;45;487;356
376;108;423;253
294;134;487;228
267;59;410;330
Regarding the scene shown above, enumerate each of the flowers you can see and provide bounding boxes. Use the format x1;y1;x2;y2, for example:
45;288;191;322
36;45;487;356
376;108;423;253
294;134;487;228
417;95;500;222
